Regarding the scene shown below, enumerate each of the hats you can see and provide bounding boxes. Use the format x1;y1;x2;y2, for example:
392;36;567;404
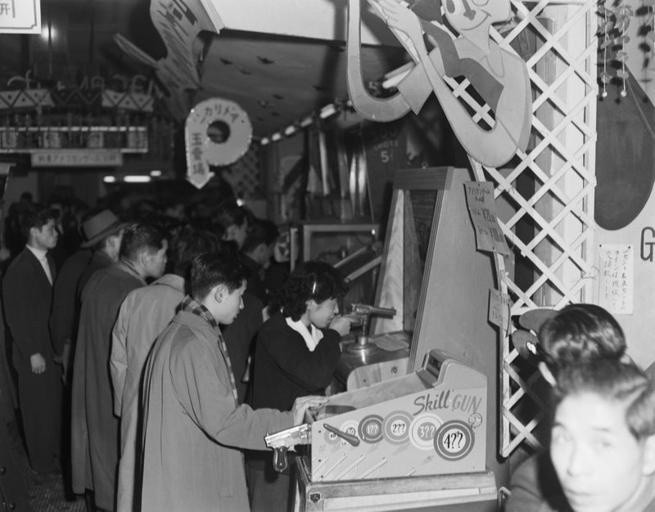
78;209;130;248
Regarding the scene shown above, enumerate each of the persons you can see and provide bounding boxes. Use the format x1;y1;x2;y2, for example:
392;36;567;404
547;362;655;512
502;303;625;512
0;188;360;511
